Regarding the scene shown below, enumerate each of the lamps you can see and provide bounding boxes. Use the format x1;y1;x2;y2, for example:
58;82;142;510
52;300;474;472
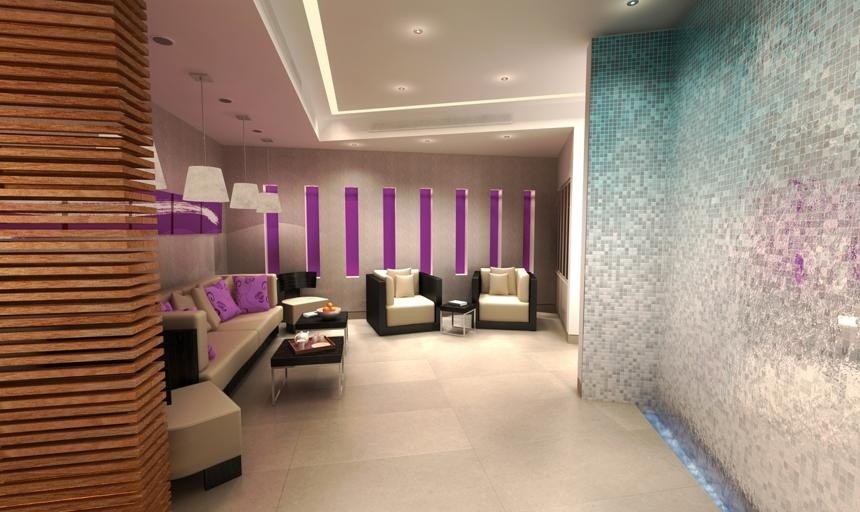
92;131;169;193
181;71;230;203
228;114;264;210
256;138;282;215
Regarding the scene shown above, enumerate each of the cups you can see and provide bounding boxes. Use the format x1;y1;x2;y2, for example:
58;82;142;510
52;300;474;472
296;340;305;350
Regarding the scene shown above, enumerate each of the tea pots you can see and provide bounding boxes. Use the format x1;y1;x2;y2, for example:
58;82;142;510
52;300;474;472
294;331;309;344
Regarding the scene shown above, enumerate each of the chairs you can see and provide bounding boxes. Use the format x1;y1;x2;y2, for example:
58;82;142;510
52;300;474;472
155;327;244;502
277;271;330;334
471;267;538;332
365;268;443;337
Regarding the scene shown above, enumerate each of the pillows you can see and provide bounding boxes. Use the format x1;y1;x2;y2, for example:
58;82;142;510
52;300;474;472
395;274;416;298
488;273;509;296
489;266;517;295
161;275;270;360
386;268;412;297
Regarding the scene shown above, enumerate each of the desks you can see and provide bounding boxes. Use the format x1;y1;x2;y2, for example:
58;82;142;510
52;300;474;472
295;311;350;356
270;336;346;407
440;303;477;337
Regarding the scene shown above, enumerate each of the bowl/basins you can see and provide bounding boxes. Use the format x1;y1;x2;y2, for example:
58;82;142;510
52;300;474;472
316;307;342;319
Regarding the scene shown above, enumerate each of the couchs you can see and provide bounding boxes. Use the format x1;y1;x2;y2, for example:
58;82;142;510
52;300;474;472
156;273;284;402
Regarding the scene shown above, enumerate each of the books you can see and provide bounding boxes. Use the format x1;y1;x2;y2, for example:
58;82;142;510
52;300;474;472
445;304;470;309
449;299;467;307
